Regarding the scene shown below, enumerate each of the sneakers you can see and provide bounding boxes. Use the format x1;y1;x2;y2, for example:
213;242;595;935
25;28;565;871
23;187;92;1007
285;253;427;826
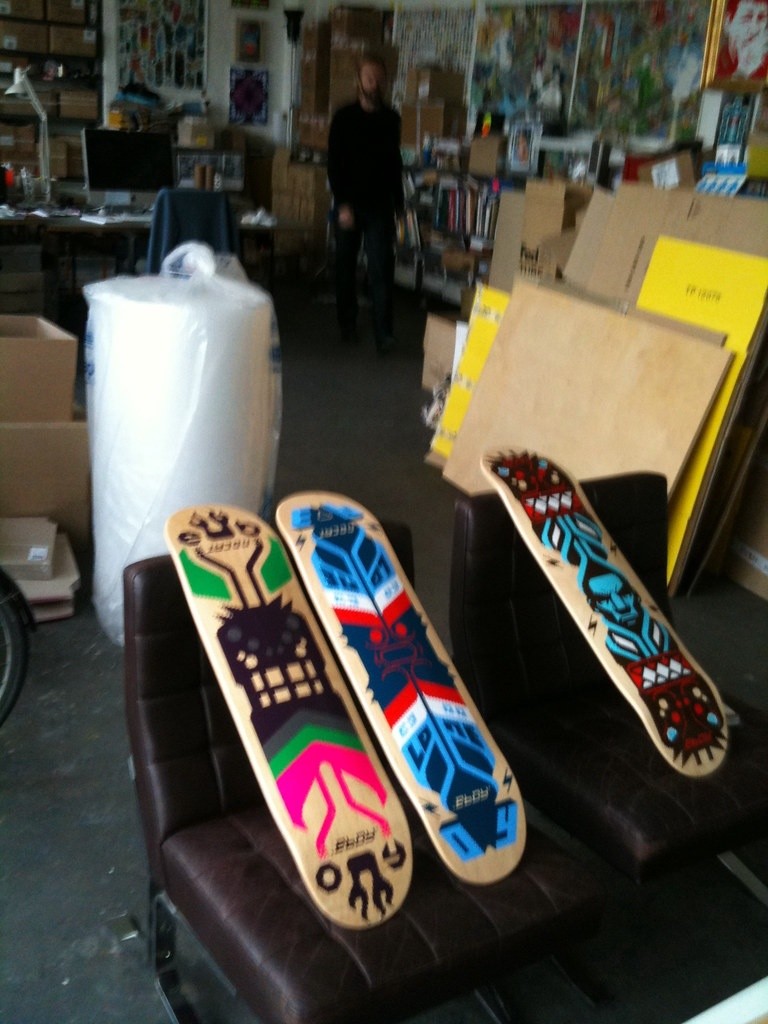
375;335;393;354
341;327;356;345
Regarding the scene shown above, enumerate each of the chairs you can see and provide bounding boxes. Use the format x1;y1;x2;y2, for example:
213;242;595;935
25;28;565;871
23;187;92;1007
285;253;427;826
145;188;241;283
122;520;640;1024
453;473;768;1012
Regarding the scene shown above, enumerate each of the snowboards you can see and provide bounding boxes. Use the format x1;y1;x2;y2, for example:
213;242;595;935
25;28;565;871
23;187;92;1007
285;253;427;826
274;488;528;886
162;500;415;932
477;443;731;781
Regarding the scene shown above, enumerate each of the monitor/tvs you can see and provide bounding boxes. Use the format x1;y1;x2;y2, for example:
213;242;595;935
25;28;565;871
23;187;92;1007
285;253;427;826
81;127;175;216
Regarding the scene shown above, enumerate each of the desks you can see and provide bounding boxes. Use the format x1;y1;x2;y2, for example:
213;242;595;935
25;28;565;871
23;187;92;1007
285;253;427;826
42;204;277;293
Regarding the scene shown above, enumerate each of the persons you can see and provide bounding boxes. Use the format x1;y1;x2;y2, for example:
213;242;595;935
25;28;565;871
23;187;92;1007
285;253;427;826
323;53;408;357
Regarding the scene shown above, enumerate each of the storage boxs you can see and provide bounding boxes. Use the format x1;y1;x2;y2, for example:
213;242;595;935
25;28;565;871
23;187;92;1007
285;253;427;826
0;1;114;579
266;8;703;392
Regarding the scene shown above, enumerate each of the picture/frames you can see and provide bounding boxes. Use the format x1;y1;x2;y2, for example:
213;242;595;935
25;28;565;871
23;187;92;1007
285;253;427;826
233;18;266;65
700;0;768;93
